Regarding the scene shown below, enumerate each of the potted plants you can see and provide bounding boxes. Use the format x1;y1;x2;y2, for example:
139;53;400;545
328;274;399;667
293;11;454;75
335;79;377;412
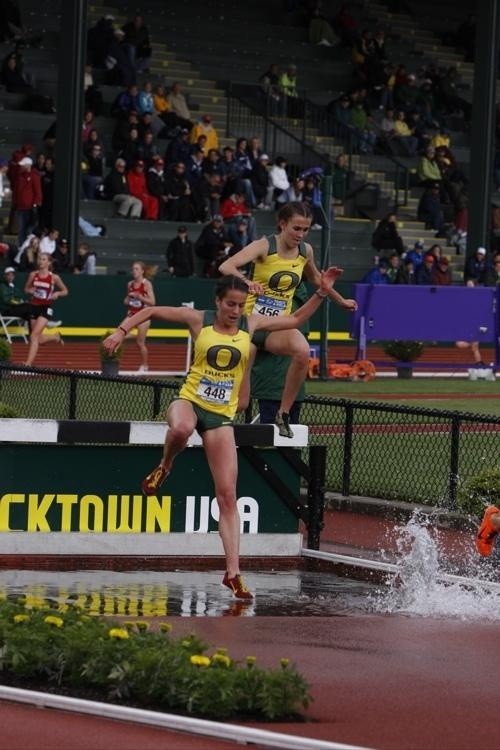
100;332;122;378
380;335;421;377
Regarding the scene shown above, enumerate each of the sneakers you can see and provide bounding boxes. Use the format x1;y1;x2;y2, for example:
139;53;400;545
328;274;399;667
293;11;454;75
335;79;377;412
274;411;293;438
56;332;64;346
140;466;170;495
138;365;148;371
222;572;253;599
230;601;253;616
476;507;500;556
47;321;62;328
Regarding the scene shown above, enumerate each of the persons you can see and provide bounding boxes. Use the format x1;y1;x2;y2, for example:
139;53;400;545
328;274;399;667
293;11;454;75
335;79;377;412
2;1;498;372
247;280;313;423
475;504;500;558
100;263;344;601
217;200;360;441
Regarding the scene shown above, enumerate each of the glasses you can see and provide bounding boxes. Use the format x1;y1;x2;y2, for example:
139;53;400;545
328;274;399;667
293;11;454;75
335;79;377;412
239;220;247;225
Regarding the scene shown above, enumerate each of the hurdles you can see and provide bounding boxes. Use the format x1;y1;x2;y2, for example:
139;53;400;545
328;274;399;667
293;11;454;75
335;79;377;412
0;417;324;556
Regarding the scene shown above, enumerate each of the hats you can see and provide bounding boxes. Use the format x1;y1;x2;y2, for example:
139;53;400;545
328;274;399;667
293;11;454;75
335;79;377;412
154;159;165;166
415;242;424;249
177;226;186;232
380;261;386;268
439;257;449;266
134;160;144;167
259;153;269;161
203;115;211;123
425;256;434;263
4;267;15;274
17;157;33;166
477;247;486;256
213;214;223;223
57;237;70;245
